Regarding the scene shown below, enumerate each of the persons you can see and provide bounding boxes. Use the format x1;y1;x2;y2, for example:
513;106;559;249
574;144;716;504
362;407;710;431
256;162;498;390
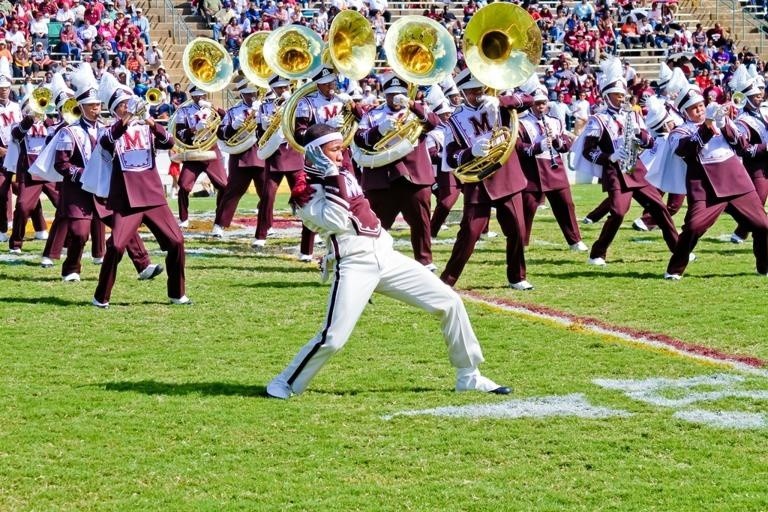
2;2;768;309
265;123;512;401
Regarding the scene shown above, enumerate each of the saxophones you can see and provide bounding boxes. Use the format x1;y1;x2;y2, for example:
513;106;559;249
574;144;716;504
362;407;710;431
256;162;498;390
619;108;639;173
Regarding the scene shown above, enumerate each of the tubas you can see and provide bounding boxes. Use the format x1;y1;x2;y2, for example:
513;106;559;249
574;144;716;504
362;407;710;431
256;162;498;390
257;24;328;157
279;9;378;157
169;37;235;163
347;14;459;169
216;30;282;154
453;0;545;181
320;40;336;72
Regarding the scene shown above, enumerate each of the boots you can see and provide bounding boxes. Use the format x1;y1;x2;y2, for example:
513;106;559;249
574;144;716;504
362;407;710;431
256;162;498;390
454;365;512;395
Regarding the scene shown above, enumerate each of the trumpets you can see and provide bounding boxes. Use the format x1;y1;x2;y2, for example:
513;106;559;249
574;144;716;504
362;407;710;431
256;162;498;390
705;92;746;120
28;88;163;125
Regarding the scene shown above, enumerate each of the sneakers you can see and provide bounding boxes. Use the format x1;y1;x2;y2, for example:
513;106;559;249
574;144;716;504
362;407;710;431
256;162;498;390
39;256;54;269
250;238;265;248
62;272;80;283
0;231;22;256
507;279;534;293
136;263;164;280
569;239;607;267
265;375;294;401
91;297;111;310
168;295;192;306
423;261;439;274
34;229;50;240
91;257;105;265
175;219;225;238
298;251;312;263
632;216;650;233
729;230;744;245
662;251;698;282
582;216;592;226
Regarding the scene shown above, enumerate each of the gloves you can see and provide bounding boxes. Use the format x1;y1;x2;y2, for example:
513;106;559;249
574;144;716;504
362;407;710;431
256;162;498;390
230;117;243;129
259;114;270;131
629;119;641;136
126;94;151;120
304;143;339;177
250;100;262;112
334;91;354;104
538;122;556;153
282;90;293;101
194;99;213;131
704;99;727;129
392;93;410;107
323;114;344;129
377;118;396;136
476;94;500;114
470;138;491;158
610;145;628;165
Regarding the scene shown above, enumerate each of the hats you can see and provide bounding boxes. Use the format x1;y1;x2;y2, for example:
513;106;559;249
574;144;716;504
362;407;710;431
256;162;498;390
67;60;103;106
428;80;459;115
94;70;135;119
517;71;548;104
266;73;292;90
379;71;408;95
643;60;705;133
452;66;484;90
596;55;627;100
726;61;766;101
235;78;258;94
18;71;77;117
0;55;13;90
307;63;336;84
187;81;208;97
